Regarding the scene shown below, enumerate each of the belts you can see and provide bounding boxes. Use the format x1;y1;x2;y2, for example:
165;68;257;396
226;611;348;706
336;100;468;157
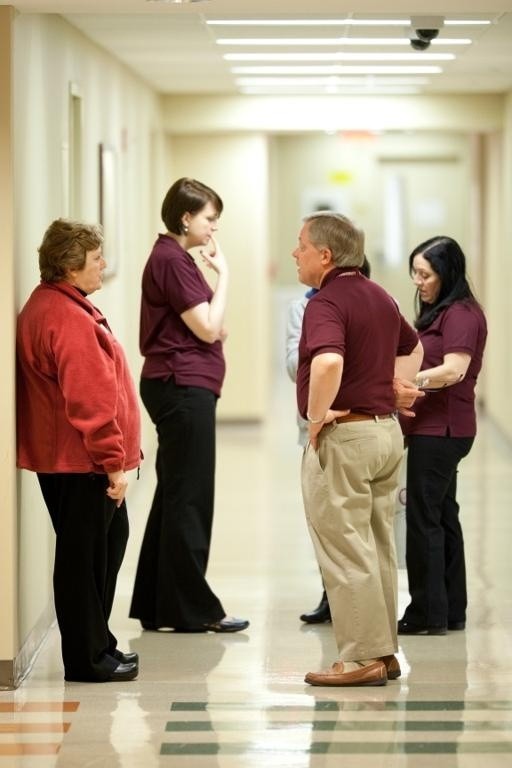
320;409;397;428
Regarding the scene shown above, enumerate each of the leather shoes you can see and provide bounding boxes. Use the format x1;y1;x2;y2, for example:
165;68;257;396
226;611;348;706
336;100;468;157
141;612;251;632
376;655;401;680
109;662;139;681
299;601;331;623
395;616;466;637
120;652;138;665
301;662;387;688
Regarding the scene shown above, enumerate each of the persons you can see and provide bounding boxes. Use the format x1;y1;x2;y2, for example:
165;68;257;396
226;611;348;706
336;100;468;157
16;216;143;683
397;235;488;633
285;255;370;623
291;211;423;686
128;176;249;633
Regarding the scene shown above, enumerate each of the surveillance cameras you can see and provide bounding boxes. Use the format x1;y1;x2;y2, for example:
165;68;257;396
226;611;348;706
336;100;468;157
410;16;443;41
409;33;431;50
